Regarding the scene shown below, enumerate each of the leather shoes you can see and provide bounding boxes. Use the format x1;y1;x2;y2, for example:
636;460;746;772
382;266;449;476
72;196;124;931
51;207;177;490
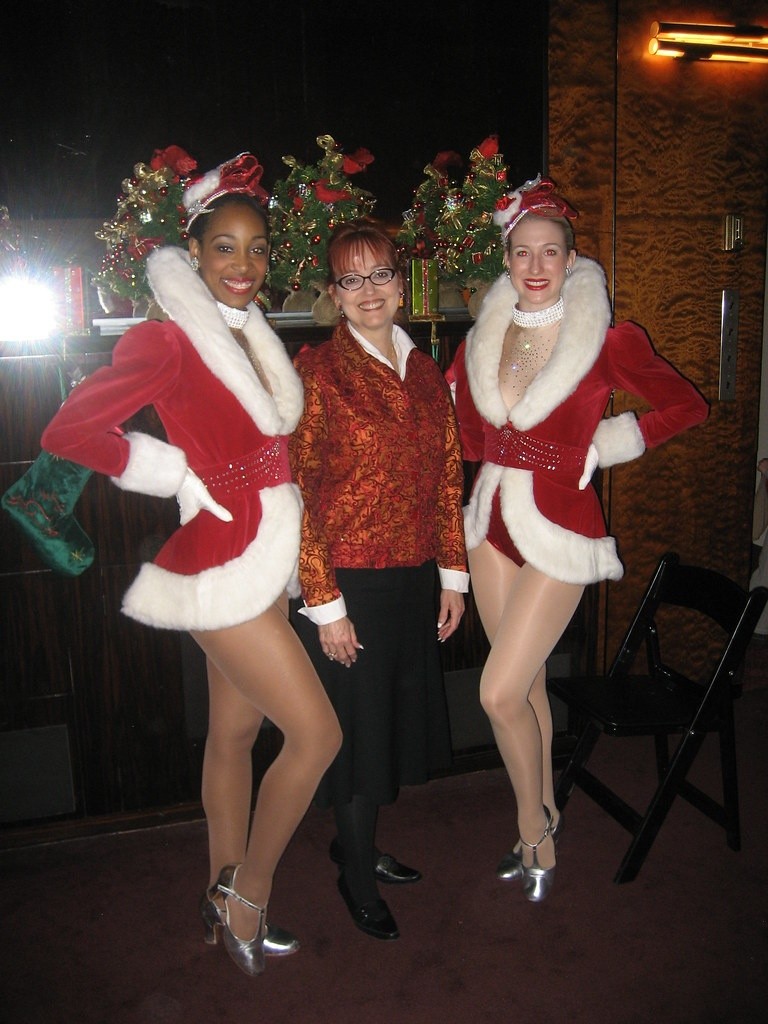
337;875;400;939
329;839;423;885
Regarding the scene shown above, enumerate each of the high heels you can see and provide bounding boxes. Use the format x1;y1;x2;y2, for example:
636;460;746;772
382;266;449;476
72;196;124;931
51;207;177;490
495;811;564;881
207;864;267;976
201;889;303;957
519;805;557;902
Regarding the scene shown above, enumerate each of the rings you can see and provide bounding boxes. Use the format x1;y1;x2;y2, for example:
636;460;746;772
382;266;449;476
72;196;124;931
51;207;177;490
329;652;336;656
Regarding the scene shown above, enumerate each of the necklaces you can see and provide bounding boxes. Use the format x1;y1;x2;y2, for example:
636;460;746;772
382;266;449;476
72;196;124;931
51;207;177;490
513;296;564;328
219;302;249;330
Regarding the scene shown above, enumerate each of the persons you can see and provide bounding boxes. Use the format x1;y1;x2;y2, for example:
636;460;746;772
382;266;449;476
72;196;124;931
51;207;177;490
40;188;345;978
443;188;710;903
286;218;470;939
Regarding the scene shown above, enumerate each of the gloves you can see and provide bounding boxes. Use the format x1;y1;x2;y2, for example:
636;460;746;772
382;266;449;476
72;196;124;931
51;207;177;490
175;468;234;525
579;444;599;490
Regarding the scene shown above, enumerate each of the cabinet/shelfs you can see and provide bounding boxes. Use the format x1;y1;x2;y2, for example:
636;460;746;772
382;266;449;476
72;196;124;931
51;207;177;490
58;312;586;825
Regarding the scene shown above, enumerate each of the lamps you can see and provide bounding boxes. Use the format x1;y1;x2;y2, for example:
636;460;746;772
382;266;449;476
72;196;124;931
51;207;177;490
645;20;768;64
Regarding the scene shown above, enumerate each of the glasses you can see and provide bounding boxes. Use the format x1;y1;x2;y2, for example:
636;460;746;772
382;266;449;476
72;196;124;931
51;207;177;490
333;267;397;291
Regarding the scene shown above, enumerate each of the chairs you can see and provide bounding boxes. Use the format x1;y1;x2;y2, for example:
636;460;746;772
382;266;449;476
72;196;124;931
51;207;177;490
546;551;768;883
